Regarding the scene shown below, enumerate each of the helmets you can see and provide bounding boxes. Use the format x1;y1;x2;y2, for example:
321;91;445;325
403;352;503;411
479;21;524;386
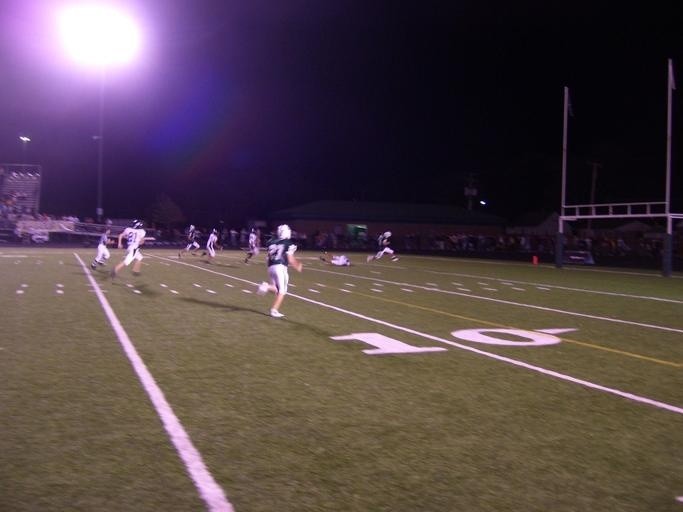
213;228;218;233
251;228;256;234
190;224;195;230
132;219;144;228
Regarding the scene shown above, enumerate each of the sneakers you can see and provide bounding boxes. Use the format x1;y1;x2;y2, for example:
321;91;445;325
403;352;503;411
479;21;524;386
391;256;399;261
255;282;269;295
270;309;285;317
367;255;372;262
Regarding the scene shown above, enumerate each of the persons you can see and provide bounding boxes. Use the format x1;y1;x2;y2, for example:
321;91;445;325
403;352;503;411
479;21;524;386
108;219;146;280
178;225;202;257
367;231;401;263
320;251;352;266
241;227;260;265
223;226;420;254
258;223;302;318
204;218;222;259
419;231;664;269
90;226;114;270
